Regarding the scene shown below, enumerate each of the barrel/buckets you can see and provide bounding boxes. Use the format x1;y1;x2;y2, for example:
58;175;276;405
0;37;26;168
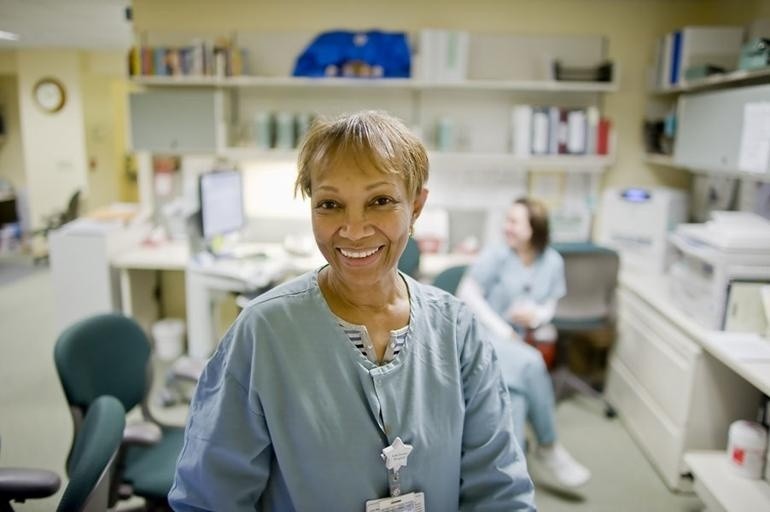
726;420;767;479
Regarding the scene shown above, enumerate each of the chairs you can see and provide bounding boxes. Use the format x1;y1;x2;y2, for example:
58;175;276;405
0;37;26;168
425;264;467;291
51;310;189;511
550;242;620;424
399;231;417;277
29;188;82;266
1;395;128;511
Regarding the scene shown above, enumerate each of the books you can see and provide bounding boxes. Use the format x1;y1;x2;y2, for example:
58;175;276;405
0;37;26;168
125;28;243;77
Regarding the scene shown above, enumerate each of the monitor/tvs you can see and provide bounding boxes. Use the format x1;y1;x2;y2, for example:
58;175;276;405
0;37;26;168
198;169;249;240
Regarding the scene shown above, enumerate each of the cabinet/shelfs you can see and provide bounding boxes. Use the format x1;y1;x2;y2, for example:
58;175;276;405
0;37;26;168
49;215;139;340
131;81;623;223
687;333;770;512
604;272;770;498
636;69;768;221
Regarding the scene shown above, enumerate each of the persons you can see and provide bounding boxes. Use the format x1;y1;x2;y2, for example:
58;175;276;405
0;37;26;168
455;196;591;493
168;111;536;512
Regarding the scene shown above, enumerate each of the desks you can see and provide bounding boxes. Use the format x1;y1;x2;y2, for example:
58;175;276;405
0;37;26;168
117;232;487;388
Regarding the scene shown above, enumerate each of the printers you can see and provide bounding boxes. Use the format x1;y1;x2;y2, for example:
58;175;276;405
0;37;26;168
665;210;770;332
